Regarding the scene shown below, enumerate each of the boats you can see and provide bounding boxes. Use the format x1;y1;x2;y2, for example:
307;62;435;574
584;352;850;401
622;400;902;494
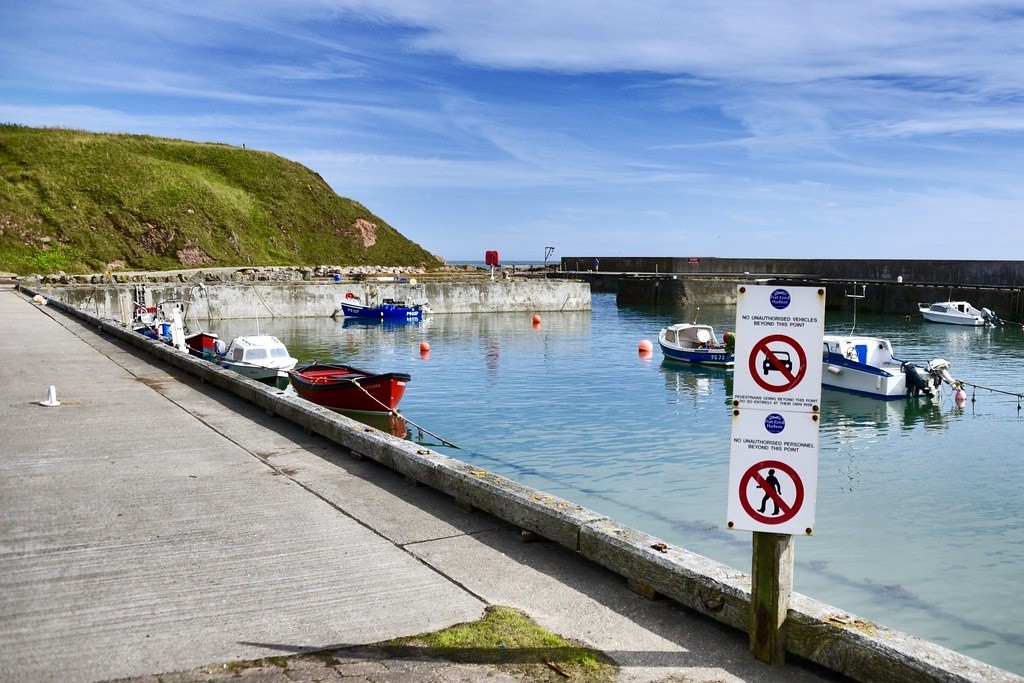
917;286;1004;326
657;306;735;366
125;285;298;391
337;284;423;318
285;358;412;416
326;407;408;439
820;285;961;402
